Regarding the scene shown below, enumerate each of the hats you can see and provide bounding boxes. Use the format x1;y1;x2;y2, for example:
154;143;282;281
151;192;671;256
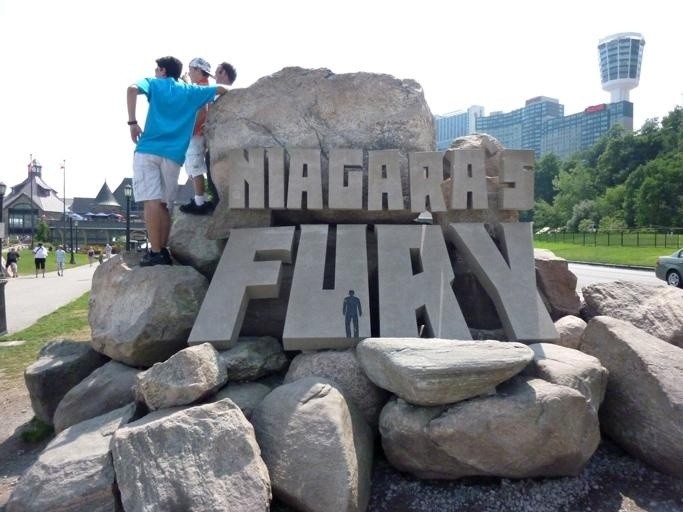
189;57;216;79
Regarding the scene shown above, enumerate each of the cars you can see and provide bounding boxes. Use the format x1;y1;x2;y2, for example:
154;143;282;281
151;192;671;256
655;247;682;291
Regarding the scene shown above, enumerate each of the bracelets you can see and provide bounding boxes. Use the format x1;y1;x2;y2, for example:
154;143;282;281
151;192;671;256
127;120;137;125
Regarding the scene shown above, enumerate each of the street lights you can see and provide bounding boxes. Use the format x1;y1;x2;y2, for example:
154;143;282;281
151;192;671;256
123;184;132;252
0;182;9;338
74;220;79;255
67;210;76;265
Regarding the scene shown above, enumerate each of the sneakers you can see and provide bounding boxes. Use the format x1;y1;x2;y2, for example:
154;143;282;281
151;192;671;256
140;246;174;268
179;195;213;215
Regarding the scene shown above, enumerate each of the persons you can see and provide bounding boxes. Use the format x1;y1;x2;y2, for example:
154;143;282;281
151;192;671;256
56;244;64;277
86;245;94;268
209;58;237;94
32;242;48;278
342;289;361;336
126;56;230;268
98;243;112;265
7;247;19;278
178;58;217;216
